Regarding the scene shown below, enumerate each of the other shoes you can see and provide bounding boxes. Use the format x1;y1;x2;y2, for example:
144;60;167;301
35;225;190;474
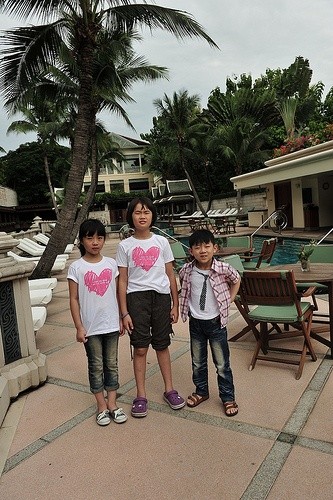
109;407;128;424
96;410;111;426
163;390;185;410
131;397;148;417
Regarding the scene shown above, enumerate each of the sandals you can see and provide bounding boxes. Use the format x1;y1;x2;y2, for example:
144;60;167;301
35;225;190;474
223;400;238;417
187;392;209;408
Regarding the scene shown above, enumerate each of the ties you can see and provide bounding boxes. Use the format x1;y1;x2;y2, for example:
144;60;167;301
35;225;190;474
196;270;209;310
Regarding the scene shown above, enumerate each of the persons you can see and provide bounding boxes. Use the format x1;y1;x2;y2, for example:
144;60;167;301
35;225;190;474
179;228;241;417
67;218;129;425
116;197;186;417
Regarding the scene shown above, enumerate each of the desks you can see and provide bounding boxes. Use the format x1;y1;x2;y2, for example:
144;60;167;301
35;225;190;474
214;247;251;257
256;263;333;358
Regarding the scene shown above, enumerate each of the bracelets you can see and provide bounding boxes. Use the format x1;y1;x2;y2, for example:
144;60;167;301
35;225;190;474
120;312;129;318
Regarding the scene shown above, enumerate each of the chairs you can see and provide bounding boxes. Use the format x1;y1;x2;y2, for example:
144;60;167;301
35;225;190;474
7;223;79;336
170;208;318;380
